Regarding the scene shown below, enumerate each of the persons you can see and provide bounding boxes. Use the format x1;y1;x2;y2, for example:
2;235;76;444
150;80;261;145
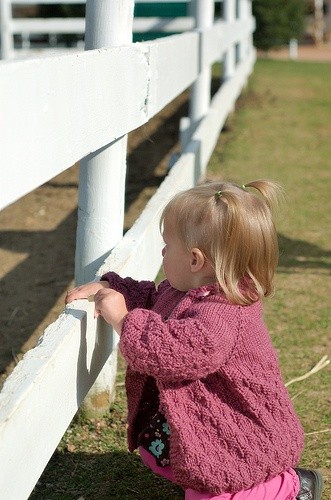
65;179;322;500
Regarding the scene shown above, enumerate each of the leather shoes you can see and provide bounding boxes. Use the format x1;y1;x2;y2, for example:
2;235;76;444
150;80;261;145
293;466;322;500
